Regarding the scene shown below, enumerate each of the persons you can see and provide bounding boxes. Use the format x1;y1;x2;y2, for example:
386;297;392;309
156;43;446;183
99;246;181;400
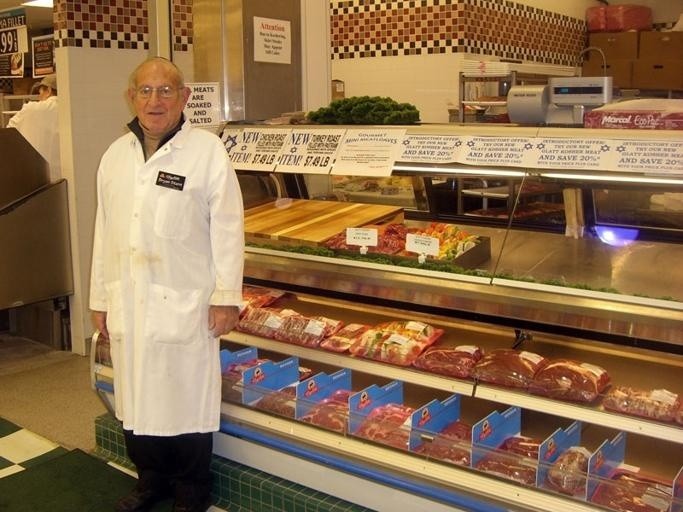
86;55;248;511
4;74;61;183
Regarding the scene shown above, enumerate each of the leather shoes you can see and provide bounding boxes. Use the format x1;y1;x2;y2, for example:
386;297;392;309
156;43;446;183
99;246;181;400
114;486;155;512
175;492;213;512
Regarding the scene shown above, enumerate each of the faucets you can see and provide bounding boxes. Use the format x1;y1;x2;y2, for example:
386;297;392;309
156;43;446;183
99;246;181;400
574;46;606;77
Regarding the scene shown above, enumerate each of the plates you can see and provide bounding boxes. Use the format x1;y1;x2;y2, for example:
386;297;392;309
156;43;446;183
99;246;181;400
458;57;577;80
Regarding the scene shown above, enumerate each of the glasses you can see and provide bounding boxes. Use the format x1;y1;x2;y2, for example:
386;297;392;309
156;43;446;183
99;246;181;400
129;86;185;98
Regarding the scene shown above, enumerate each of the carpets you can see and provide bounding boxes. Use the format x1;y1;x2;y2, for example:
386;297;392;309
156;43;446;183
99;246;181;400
0;414;173;511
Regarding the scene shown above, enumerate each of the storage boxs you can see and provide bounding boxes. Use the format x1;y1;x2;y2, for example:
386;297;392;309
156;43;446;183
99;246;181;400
631;60;682;91
588;31;637;59
639;32;682;60
583;58;632;89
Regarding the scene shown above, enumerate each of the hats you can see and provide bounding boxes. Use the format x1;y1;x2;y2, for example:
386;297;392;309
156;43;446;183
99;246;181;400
31;74;57;95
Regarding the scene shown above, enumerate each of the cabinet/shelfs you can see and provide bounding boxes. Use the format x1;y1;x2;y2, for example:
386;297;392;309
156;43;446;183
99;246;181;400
457;70;577;228
92;121;682;510
